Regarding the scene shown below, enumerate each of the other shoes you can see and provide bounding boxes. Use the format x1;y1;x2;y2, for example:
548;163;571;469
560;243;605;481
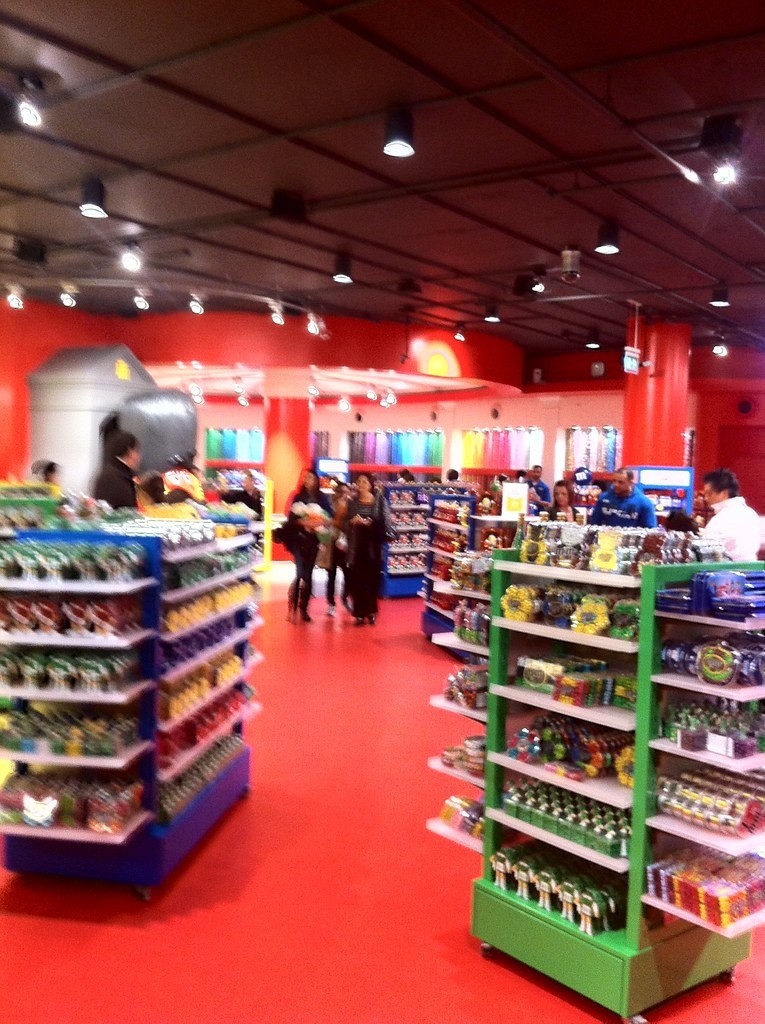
299;609;311;622
353;618;363;626
328;606;334;616
369;615;375;624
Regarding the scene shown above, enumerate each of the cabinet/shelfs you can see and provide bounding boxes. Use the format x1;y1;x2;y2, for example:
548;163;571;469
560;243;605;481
0;481;263;898
382;485;764;1023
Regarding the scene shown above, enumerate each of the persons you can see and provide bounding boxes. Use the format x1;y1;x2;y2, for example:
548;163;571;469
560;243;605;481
666;511;699;536
546;479;581;522
699;468;763;561
93;432;141;510
223;472;263;548
431;469;459;483
283;469;336;624
591;468;657;528
32;460;60;484
340;472;397;626
133;470;201;520
398;468;416;483
324;483;352;618
164;447;205;504
500;465;526;482
526;465;552;516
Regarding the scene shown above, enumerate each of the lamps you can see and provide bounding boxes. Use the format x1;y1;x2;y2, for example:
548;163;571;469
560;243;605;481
709;284;730;307
332;262;351;284
485;303;500;322
585;331;599;349
382;111;415;157
594;223;619;254
79;182;108;219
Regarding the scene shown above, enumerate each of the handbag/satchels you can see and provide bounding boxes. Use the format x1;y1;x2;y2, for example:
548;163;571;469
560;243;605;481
335;534;348;552
